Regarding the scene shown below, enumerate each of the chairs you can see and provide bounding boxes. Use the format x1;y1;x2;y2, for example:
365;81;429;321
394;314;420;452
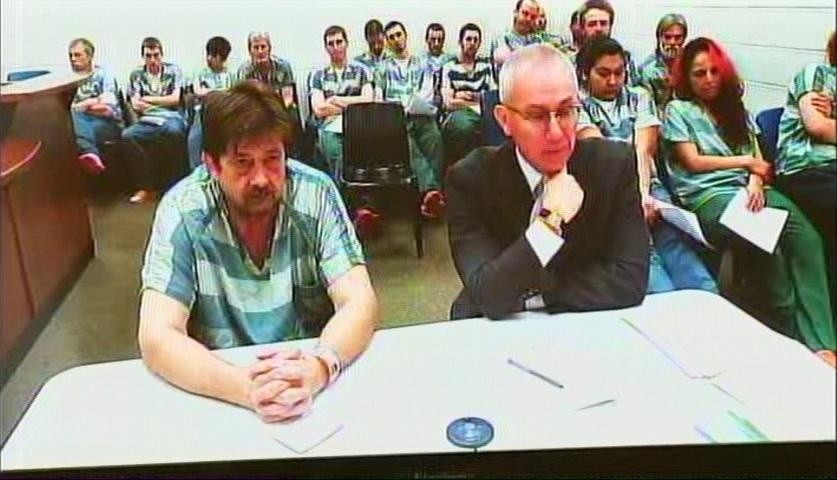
753;107;785;170
96;65;320;191
653;141;766;305
336;101;425;260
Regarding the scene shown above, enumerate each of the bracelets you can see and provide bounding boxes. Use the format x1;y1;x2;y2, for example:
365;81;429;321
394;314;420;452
305;342;342;388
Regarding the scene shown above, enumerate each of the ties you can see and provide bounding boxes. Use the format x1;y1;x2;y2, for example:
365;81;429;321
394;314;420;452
527;180;544;226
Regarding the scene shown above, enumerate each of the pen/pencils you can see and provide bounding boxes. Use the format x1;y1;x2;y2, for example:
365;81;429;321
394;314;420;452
507;358;564;388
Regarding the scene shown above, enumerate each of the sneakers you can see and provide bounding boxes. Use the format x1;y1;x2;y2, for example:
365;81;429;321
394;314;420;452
420;190;447;220
78;152;108;177
813;349;837;368
124;187;157;205
354;208;383;230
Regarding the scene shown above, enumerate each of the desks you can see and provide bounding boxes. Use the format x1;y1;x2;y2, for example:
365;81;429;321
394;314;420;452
2;284;836;479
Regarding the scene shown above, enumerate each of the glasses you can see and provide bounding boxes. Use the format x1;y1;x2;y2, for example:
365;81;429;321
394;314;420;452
502;103;583;127
662;31;685;42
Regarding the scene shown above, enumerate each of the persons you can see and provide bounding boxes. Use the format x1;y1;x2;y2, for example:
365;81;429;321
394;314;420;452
133;77;380;424
441;41;651;323
62;38;126;175
772;29;836;230
117;36;193;203
182;35;241;177
659;36;835;369
234;30;299;159
